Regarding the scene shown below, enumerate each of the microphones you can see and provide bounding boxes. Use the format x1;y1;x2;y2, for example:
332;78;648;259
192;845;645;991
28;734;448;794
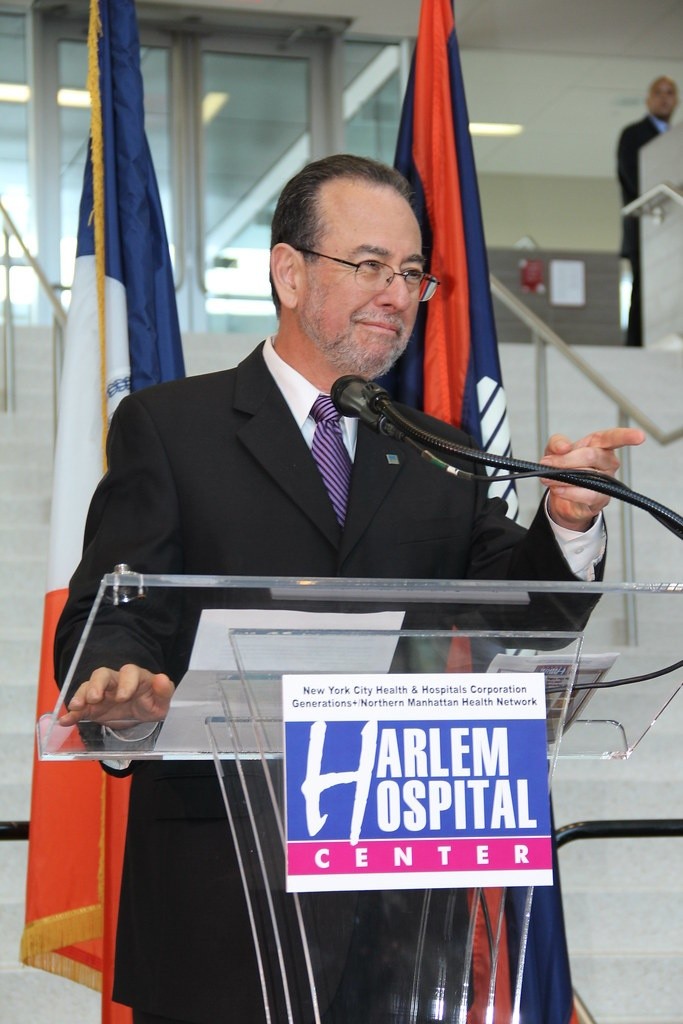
330;374;406;442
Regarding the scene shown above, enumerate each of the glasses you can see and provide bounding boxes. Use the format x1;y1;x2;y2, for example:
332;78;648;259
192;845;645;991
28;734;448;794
292;246;440;301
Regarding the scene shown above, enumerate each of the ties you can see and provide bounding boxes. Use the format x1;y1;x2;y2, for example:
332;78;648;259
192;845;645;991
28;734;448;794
309;395;352;528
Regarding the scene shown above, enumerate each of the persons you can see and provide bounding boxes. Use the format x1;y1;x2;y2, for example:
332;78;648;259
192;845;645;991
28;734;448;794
617;75;679;347
53;155;647;1023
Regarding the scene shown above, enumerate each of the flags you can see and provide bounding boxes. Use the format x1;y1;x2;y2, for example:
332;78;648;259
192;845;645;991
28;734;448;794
19;0;185;1023
376;0;581;1024
